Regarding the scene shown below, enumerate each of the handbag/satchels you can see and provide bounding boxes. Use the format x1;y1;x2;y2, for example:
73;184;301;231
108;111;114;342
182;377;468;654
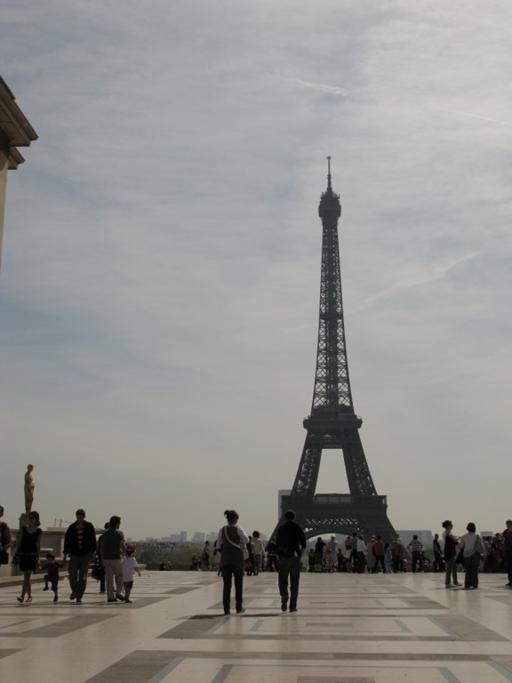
212;550;222;566
240;544;250;560
90;565;104;581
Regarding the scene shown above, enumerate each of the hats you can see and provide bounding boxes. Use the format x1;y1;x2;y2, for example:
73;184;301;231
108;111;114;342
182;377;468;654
126;543;137;553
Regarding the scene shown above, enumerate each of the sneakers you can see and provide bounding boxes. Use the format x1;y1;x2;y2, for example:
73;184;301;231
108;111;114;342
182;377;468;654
43;587;49;590
26;597;32;602
53;597;58;602
446;582;478;590
17;596;24;602
70;592;82;602
99;590;133;604
474;535;484;554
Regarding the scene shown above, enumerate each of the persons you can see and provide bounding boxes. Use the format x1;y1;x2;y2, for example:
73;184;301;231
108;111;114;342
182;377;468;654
24;464;34;514
441;520;461;589
0;506;12;564
62;508;97;602
275;511;307;612
192;530;446;576
14;511;43;603
215;510;249;614
448;533;506;574
100;516;126;602
457;522;483;590
503;519;512;587
39;553;66;602
120;543;141;604
97;522;117;593
